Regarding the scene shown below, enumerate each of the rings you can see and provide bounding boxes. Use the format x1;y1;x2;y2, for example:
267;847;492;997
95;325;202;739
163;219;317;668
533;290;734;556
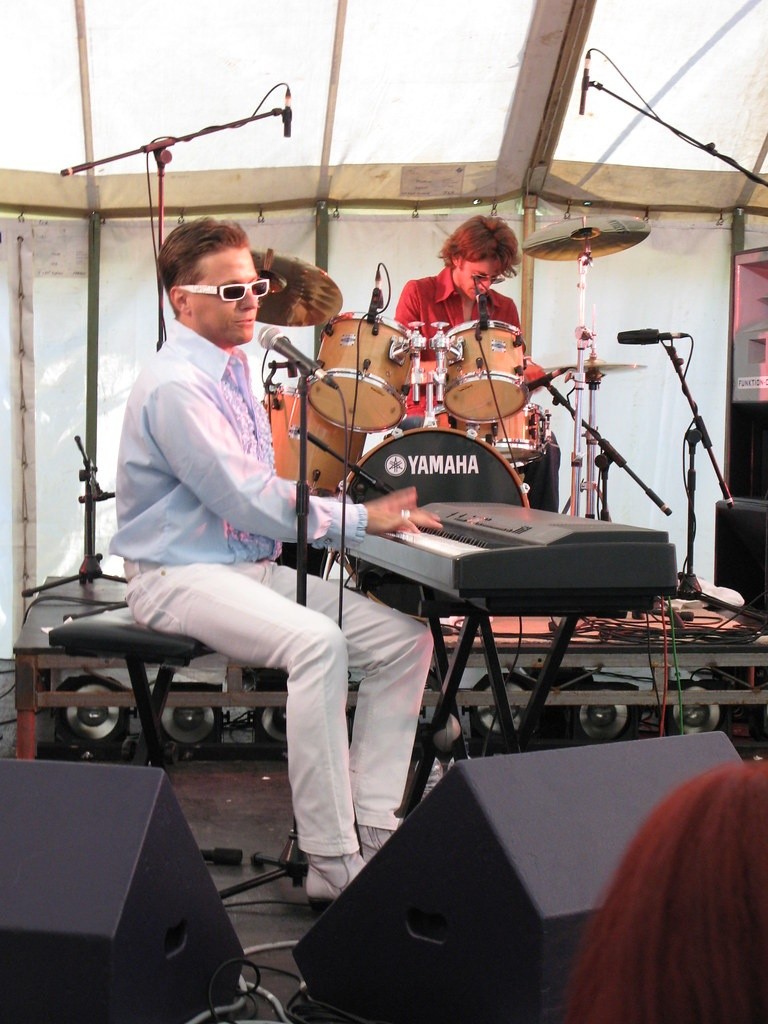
401;509;411;521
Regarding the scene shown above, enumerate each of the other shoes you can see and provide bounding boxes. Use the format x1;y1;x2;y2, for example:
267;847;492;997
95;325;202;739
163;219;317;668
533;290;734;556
306;853;369;908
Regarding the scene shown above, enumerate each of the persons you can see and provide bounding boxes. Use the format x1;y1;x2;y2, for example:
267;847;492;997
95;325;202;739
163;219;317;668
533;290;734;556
565;761;768;1024
395;216;520;416
113;218;442;914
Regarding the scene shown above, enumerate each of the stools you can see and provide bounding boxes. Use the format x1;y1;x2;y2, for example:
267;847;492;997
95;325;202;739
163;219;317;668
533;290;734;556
50;606;244;866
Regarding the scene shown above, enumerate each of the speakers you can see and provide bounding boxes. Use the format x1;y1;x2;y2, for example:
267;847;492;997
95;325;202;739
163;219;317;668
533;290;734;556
291;731;743;1024
0;758;245;1024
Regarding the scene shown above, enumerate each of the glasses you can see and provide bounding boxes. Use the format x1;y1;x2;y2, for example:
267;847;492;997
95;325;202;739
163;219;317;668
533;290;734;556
468;270;513;286
167;277;274;303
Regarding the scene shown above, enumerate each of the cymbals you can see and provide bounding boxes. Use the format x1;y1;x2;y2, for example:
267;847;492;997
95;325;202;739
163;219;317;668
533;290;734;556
522;213;652;261
248;249;343;327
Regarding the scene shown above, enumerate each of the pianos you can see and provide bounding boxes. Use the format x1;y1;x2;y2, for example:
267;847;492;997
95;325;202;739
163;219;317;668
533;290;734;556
343;501;679;826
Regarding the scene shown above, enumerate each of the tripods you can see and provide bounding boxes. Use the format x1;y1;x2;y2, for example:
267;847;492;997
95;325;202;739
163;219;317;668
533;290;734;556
652;339;768;628
21;108;283;604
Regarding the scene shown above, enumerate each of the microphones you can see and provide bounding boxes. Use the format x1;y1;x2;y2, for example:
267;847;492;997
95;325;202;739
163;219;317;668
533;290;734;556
284;88;292;137
580;53;591;115
618;329;691;345
527;367;570;391
258;326;339;388
375;270;384;309
474;290;490;318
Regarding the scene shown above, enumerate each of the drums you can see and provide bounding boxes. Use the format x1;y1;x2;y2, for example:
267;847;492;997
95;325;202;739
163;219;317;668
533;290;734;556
443;317;532;424
454;402;545;470
336;426;531;625
263;386;367;497
305;311;416;434
